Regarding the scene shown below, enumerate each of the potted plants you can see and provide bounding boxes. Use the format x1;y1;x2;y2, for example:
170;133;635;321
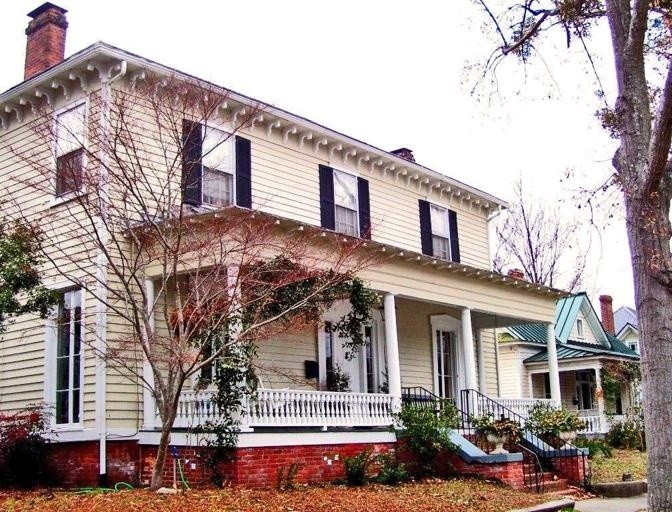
468;411;525;455
526;403;587;450
